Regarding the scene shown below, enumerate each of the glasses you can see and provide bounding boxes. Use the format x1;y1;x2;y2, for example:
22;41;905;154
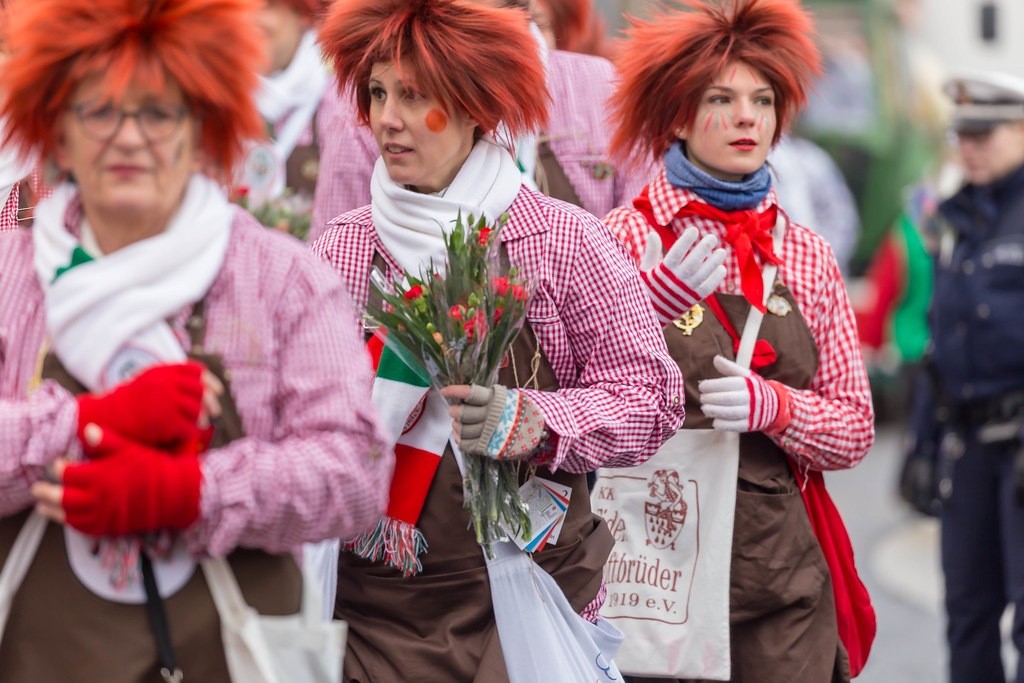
67;100;194;139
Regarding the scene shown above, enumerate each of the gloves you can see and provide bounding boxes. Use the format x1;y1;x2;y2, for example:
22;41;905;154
699;354;778;432
640;225;726;327
900;438;943;517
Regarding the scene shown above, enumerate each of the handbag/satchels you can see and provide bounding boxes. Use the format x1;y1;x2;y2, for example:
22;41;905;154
200;553;348;682
590;428;737;679
785;451;878;680
486;540;622;679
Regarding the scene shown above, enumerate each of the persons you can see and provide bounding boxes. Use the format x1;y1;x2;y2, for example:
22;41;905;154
0;1;392;683
1;1;941;422
900;75;1023;683
604;1;876;683
306;1;686;683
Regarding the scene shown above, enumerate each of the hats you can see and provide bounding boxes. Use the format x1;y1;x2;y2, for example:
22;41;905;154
941;69;1024;129
